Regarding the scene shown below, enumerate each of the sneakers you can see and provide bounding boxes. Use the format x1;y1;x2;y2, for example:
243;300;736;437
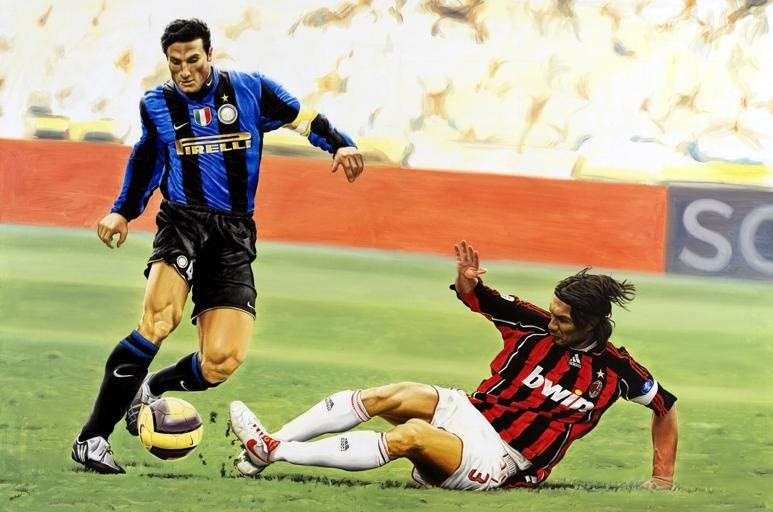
229;400;281;478
72;432;126;474
125;372;160;436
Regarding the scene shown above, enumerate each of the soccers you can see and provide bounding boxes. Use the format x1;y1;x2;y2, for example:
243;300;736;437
137;396;202;461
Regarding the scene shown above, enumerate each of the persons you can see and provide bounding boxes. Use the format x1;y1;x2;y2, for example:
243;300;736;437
230;239;678;490
72;18;364;474
